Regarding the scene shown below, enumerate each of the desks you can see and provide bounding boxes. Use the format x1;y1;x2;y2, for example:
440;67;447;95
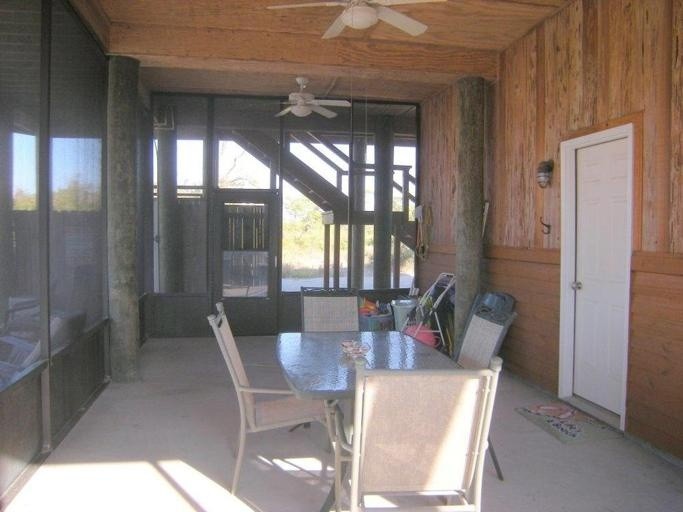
274;330;497;511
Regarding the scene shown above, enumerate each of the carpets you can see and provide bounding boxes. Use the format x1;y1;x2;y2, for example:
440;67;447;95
513;397;626;445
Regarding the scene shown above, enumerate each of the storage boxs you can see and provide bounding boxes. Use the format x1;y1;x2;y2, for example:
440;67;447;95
391;299;419;331
358;313;395;333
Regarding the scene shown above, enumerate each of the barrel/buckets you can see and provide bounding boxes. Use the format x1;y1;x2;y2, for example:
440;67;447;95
391;301;416;332
359;303;394;331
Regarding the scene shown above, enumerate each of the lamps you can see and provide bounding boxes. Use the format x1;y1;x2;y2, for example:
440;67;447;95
291;104;312;117
535;160;553;189
341;5;378;29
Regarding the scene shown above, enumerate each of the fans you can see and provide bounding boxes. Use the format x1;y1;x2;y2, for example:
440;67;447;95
248;76;354;120
264;0;437;44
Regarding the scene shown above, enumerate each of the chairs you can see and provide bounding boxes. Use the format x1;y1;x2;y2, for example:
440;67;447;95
299;286;362;332
451;291;518;482
206;299;347;498
335;356;504;511
